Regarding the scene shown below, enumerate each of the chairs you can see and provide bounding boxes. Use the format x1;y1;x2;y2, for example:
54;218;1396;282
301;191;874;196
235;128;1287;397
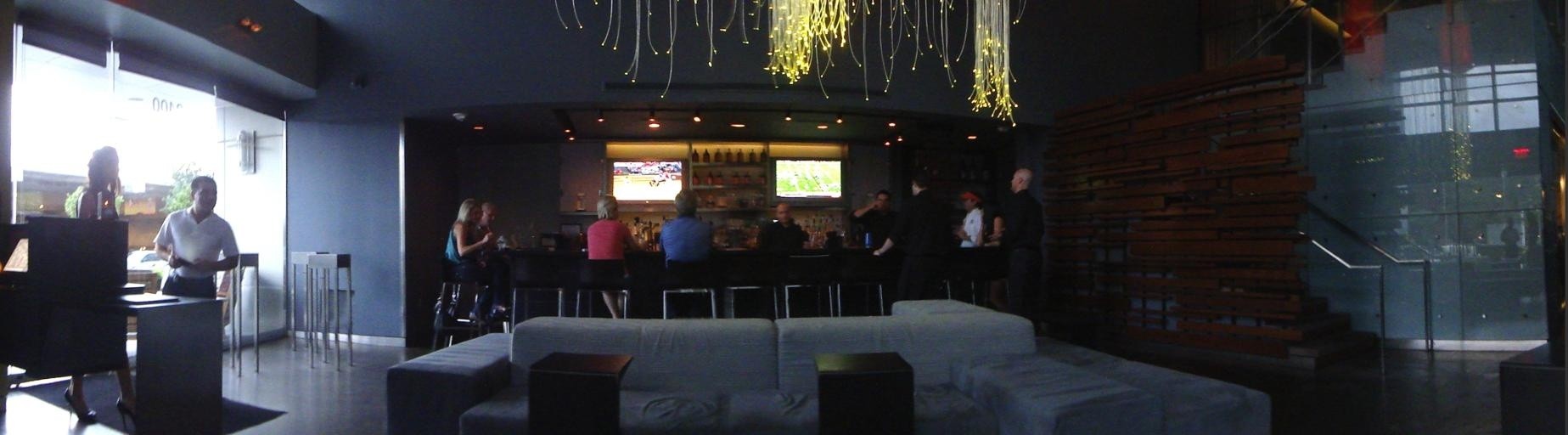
433;248;1010;347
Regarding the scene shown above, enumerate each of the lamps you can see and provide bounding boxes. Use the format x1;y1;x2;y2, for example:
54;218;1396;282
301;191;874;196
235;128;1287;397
237;128;256;173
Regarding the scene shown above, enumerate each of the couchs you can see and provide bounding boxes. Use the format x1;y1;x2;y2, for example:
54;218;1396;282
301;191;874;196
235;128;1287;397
384;299;1270;435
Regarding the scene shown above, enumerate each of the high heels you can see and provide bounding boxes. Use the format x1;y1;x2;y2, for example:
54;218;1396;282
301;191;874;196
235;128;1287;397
63;387;97;422
116;398;137;430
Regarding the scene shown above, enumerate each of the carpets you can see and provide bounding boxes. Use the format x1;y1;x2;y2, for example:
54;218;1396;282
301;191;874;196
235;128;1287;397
14;369;288;435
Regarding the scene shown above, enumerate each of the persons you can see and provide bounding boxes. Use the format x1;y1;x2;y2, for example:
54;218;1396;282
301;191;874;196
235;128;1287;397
441;199;509;326
659;188;714;319
988;172;1043;320
471;201;496;249
64;146;136;432
580;195;648;318
755;204;811;249
153;176;240;299
873;174;951;316
1500;217;1519;257
851;190;901;247
953;190;990;249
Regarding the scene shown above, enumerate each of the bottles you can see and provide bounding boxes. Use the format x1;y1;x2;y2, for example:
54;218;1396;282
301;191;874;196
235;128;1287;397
811;218;829;232
576;193;586;211
693;170;766;184
692;148;768;163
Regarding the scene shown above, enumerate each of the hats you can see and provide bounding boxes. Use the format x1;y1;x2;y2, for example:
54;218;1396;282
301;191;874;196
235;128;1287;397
960;191;980;202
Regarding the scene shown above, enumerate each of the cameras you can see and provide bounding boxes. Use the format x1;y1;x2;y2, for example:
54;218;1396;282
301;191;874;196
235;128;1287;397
176;257;192;266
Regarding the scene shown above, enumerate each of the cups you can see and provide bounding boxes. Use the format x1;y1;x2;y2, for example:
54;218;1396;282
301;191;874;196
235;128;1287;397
864;232;874;249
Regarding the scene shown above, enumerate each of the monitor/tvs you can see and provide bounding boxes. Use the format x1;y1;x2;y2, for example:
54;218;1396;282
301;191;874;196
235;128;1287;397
607;162;685;206
771;158;848;205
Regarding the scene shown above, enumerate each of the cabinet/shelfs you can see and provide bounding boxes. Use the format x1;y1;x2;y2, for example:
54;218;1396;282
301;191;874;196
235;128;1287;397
690;141;770;214
212;250;353;378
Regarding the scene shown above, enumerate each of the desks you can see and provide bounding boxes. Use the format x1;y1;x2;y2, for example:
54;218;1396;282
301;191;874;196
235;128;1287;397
124;287;223;423
